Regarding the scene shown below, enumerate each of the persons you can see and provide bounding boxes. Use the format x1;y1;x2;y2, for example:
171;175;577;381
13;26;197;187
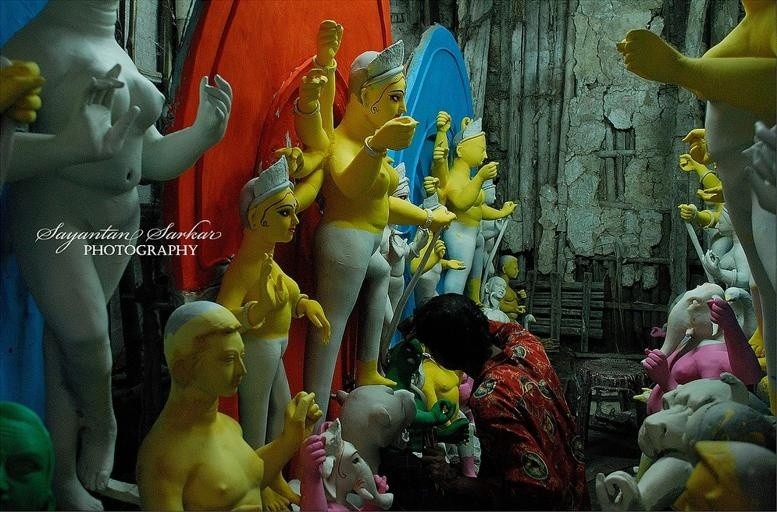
589;0;777;511
0;2;536;512
397;291;593;510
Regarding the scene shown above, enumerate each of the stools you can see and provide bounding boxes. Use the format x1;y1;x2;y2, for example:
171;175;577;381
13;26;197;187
574;358;647;449
